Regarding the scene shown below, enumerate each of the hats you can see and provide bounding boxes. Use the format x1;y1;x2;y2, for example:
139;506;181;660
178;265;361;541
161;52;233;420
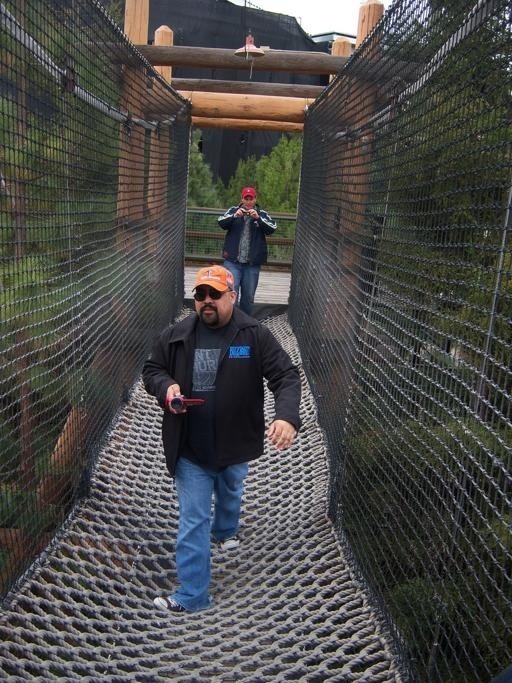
242;186;256;197
192;264;235;291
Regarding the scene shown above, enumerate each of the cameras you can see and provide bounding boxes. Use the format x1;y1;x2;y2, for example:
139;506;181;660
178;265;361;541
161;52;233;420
242;211;250;216
171;397;206;410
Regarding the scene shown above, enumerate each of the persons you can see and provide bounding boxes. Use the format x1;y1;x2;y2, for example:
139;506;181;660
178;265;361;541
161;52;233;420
215;186;278;314
142;263;303;612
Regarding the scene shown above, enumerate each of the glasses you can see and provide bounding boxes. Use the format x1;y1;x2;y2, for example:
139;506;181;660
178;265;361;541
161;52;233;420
194;288;222;301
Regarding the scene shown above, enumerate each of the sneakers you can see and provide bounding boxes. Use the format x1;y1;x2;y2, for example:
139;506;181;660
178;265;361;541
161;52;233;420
154;596;186;612
221;535;240;552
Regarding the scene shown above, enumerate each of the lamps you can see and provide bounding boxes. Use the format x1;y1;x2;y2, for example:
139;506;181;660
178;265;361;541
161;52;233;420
234;26;266;62
